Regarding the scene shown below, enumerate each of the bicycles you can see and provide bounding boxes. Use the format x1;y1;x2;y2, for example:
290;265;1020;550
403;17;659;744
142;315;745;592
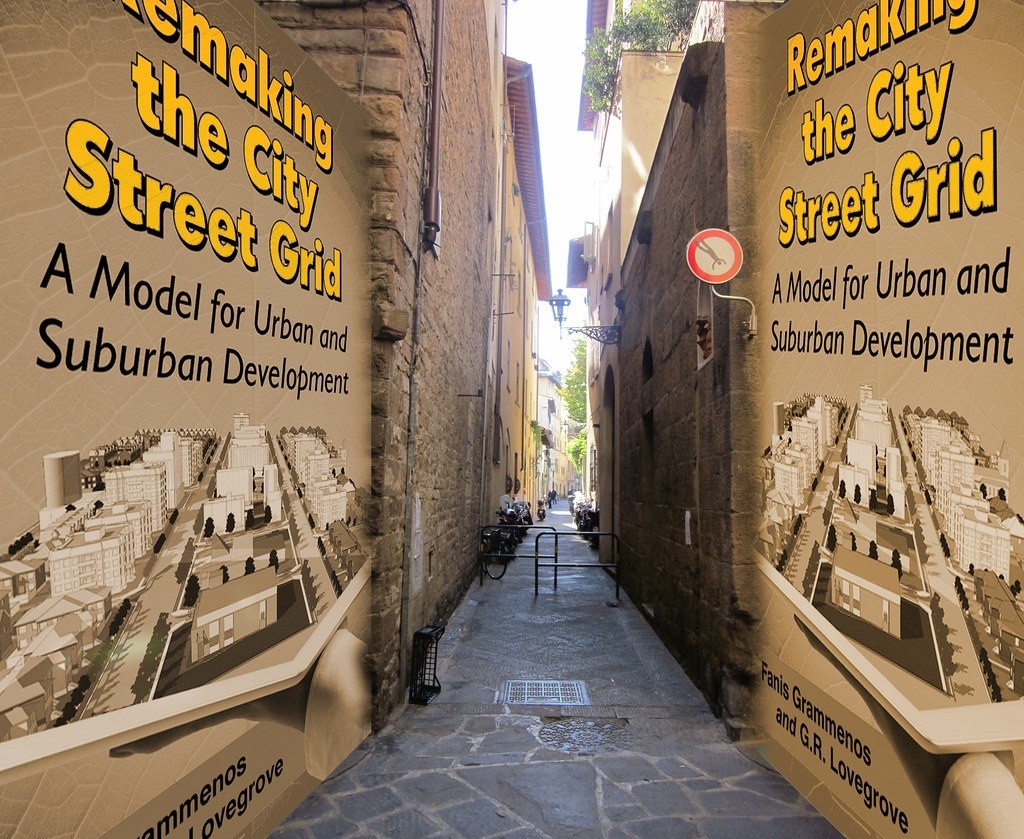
477;525;508;580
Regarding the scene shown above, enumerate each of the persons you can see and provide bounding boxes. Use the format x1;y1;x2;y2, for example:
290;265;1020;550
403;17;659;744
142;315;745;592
546;489;559;509
568;489;573;495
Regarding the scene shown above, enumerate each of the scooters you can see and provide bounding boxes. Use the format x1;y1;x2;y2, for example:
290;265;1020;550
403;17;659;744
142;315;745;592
537;494;547;521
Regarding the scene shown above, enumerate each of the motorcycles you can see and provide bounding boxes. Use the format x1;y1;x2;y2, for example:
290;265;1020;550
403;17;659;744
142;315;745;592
567;491;599;545
489;497;533;564
